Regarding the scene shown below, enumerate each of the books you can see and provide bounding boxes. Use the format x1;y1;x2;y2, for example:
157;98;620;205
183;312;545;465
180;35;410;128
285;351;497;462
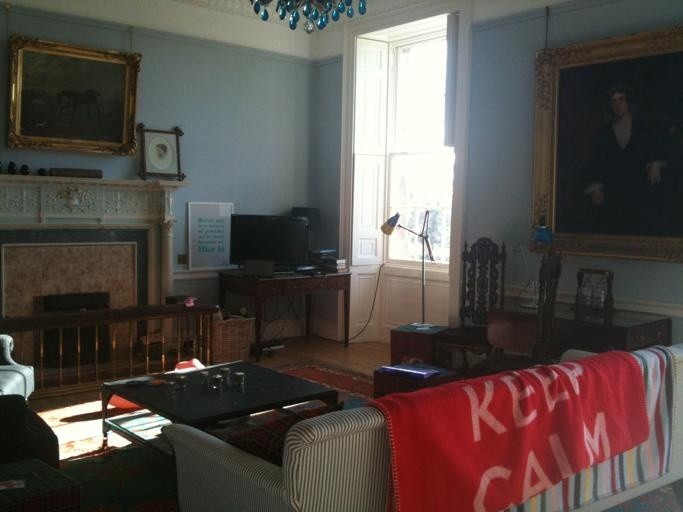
321;256;349;273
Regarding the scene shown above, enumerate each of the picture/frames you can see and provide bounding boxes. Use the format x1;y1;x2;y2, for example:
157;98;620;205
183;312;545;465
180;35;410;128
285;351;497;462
6;31;144;156
135;122;186;181
574;267;614;315
530;23;683;264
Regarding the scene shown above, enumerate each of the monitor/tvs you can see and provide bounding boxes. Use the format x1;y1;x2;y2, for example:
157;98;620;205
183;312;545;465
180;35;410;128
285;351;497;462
230;214;309;275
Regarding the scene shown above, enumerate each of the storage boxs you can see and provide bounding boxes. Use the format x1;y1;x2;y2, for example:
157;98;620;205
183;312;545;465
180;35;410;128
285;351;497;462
390;322;450;367
432;324;488;371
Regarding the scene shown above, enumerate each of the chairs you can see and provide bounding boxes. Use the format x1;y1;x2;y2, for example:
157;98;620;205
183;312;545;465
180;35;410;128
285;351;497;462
459;237;505;327
487;250;563;356
0;334;35;405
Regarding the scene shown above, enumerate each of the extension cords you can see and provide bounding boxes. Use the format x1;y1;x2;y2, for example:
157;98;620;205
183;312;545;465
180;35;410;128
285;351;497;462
262;344;285;351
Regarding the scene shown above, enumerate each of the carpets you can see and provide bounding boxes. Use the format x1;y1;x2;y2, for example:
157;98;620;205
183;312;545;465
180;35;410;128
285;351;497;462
57;358;377;512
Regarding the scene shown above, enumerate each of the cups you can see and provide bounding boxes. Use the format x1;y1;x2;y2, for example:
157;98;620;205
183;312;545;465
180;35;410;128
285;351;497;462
162;366;246;393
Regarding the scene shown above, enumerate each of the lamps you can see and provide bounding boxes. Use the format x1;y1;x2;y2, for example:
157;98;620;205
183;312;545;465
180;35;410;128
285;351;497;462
529;216;562;254
382;210;434;324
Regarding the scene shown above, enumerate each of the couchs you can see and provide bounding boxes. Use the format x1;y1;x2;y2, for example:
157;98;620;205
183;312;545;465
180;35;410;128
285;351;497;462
160;345;683;512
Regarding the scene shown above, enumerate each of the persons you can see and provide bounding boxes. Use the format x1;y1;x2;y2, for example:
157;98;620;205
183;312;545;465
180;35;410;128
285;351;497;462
581;82;672;235
154;144;171;168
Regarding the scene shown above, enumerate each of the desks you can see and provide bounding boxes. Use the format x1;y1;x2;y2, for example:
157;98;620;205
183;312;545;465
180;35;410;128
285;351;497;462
214;267;352;363
0;457;81;511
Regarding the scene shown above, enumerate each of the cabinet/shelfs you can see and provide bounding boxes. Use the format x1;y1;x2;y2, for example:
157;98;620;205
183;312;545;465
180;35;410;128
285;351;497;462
486;294;673;372
